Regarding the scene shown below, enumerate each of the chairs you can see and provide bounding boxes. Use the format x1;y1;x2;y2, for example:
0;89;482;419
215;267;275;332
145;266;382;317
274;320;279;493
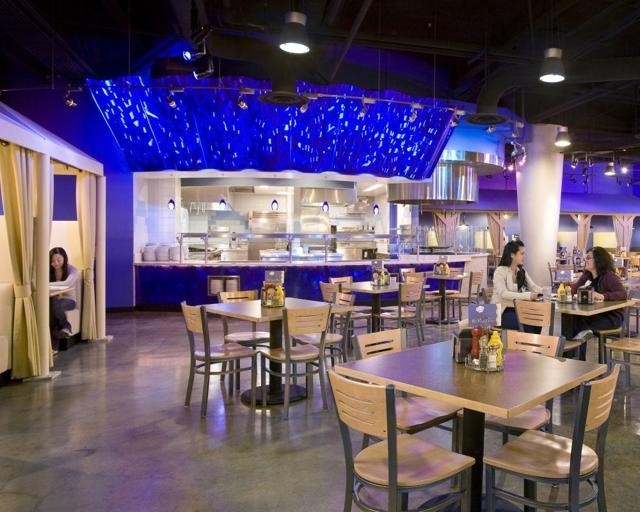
354;325;460;490
287;291;357;386
215;288;273;389
481;362;624;512
325;371;478;511
316;253;639;413
259;304;331;417
483;325;564;500
180;300;260;418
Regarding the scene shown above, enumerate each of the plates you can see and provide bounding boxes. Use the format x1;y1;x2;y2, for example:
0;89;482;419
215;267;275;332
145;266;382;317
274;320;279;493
142;244;189;261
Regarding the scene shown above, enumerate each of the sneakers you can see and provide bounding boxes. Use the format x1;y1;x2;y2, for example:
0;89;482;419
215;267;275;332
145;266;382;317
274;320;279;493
573;330;593;344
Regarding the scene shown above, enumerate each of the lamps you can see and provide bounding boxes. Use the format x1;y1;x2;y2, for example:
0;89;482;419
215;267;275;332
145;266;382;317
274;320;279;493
62;0;629;177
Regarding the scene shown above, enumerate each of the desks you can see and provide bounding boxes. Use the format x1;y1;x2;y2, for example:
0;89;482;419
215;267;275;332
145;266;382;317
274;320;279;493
195;294;356;408
335;334;608;508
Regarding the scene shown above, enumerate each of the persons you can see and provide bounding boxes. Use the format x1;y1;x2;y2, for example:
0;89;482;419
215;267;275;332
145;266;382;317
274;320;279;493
490;240;543;334
558;246;627;361
49;247;78;355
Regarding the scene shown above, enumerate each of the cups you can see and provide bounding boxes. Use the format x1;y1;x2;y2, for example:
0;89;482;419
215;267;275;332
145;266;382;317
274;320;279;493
542;285;552;302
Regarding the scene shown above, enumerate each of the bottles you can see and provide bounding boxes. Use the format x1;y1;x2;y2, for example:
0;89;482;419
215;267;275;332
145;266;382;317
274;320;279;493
261;281;285;308
470;327;504;371
433;262;450;275
619;250;628;258
371;267;391;286
557;283;572;304
